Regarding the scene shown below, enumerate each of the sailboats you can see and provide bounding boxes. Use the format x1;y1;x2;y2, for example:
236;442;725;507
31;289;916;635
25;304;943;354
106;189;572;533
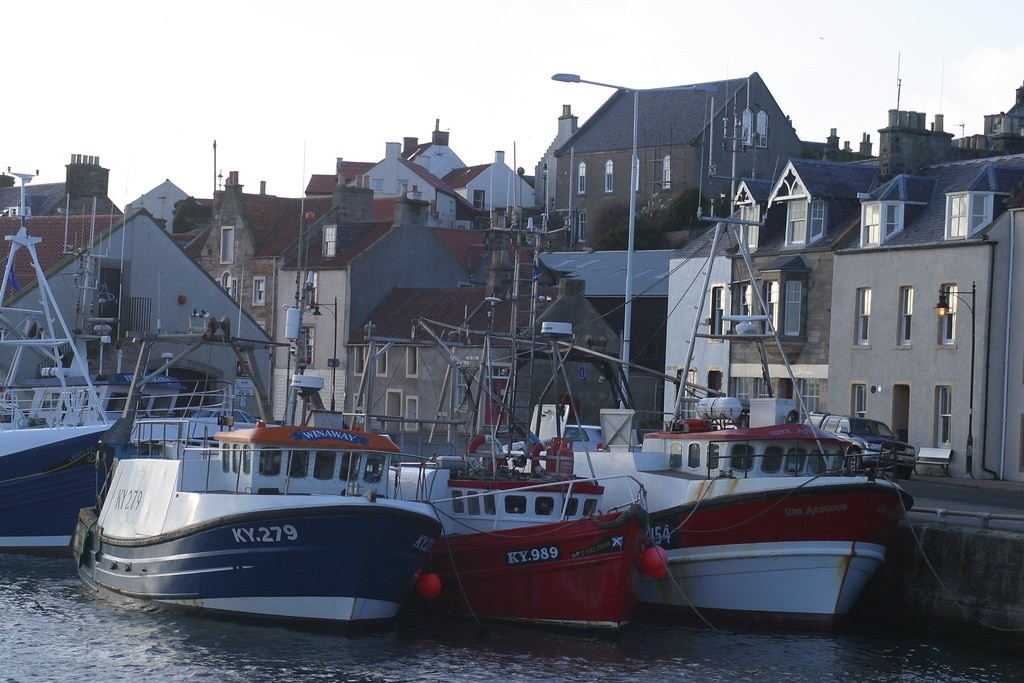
0;217;914;639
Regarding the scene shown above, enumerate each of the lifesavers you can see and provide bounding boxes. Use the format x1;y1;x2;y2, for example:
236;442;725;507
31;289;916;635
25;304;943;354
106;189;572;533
466;434;505;474
530;436;560;478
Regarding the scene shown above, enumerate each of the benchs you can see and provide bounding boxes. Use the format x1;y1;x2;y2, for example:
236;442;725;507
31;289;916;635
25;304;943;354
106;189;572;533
913;448;954;479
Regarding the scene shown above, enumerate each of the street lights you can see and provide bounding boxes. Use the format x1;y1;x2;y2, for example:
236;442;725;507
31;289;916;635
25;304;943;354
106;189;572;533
552;73;639;412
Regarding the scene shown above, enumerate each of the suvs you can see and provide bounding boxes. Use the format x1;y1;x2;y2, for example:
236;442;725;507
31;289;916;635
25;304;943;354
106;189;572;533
802;412;916;481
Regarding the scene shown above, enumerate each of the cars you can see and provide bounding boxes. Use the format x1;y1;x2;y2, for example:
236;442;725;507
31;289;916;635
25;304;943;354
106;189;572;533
501;424;604;454
